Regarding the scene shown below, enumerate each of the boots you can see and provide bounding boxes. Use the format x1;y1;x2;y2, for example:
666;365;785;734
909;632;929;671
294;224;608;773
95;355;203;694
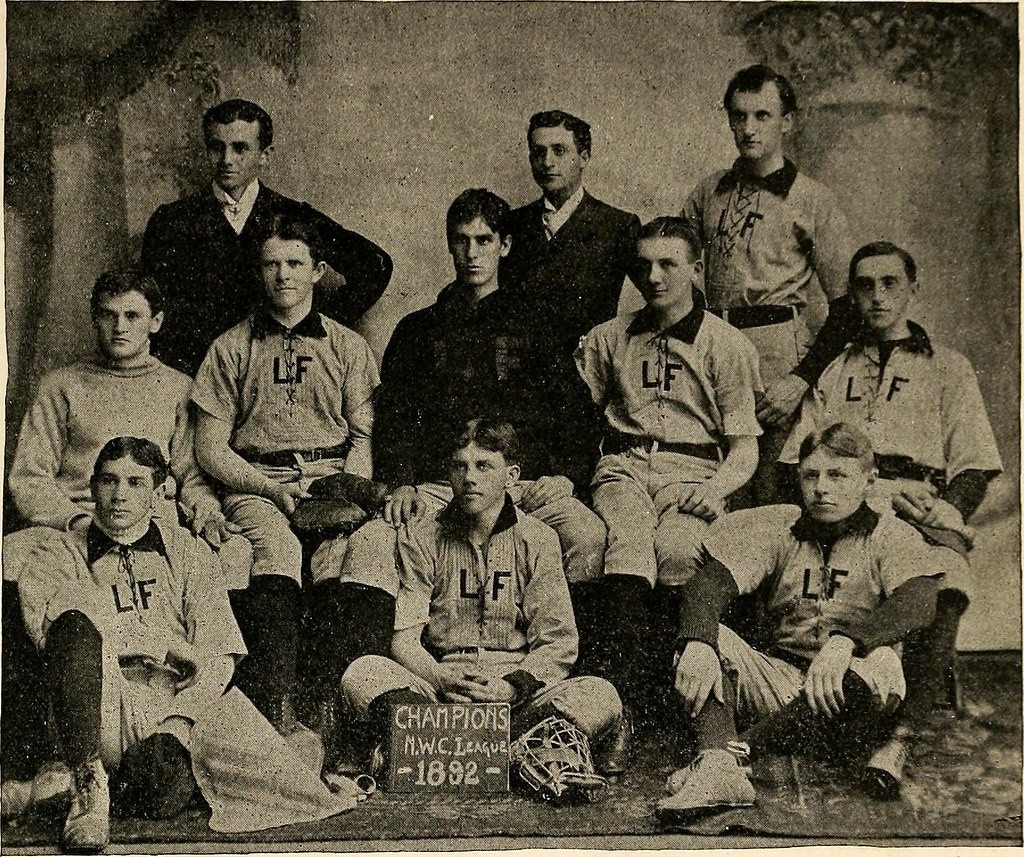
311;700;363;775
267;692;298;738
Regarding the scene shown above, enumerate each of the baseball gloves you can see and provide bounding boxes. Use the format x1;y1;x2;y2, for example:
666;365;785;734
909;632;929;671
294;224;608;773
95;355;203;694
291;472;389;538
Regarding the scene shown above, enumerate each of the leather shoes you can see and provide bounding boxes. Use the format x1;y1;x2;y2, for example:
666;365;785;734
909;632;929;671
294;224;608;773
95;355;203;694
599;715;631;773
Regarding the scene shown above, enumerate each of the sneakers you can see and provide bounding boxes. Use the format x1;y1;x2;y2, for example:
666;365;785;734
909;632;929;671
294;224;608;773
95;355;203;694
2;780;34;817
866;726;920;801
655;749;756;823
33;760;70;809
666;751;706;795
62;759;110;850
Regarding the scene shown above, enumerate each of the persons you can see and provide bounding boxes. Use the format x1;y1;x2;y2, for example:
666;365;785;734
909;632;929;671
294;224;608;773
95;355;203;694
0;61;1006;852
140;98;392;380
